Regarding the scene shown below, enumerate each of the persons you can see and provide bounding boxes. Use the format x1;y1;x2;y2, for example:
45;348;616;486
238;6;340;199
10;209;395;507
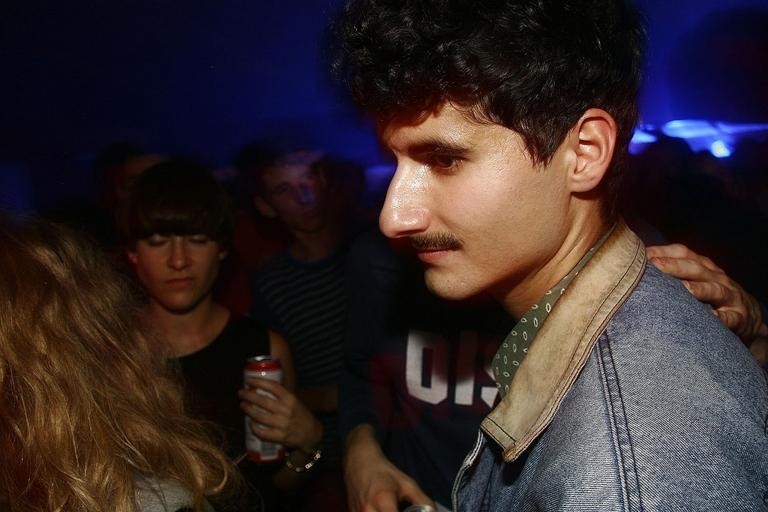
325;1;766;510
0;218;259;511
94;126;768;509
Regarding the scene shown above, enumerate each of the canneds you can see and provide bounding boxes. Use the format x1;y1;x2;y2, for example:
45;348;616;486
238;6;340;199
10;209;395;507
240;355;286;463
404;504;434;512
